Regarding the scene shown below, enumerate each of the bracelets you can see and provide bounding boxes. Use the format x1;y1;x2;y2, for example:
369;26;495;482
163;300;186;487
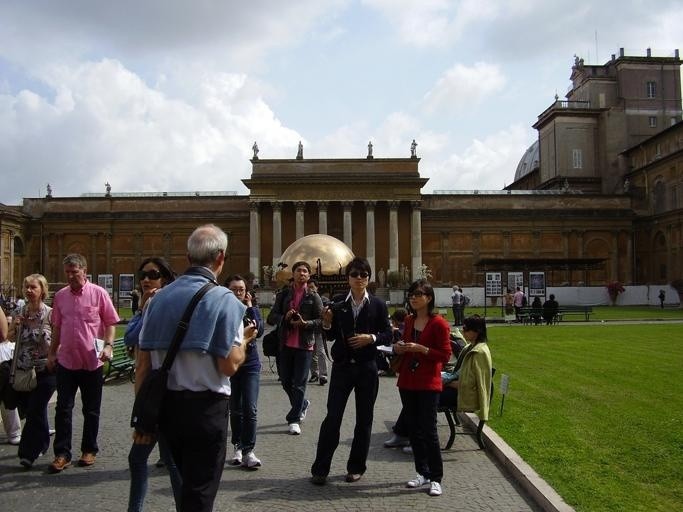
104;342;114;349
425;347;430;354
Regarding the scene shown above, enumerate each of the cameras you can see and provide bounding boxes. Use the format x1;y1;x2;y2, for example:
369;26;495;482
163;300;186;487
323;301;352;313
291;312;304;320
243;317;255;328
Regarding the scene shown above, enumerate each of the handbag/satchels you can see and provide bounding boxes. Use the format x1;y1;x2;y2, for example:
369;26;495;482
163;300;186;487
12;367;38;391
390;352;404;371
131;370;168;432
263;331;279;356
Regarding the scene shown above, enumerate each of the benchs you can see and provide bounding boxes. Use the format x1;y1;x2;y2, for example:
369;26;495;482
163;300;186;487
441;368;496;451
557;306;592;322
517;306;561;327
101;338;151;383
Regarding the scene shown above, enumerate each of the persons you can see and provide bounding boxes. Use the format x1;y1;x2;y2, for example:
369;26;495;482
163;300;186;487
266;261;322;434
383;312;492;446
220;274;264;472
238;280;408;384
6;272;58;468
133;223;258;510
0;304;22;444
311;258;393;486
394;277;452;497
451;283;561;326
47;252;119;471
0;279;25;326
122;258;177;510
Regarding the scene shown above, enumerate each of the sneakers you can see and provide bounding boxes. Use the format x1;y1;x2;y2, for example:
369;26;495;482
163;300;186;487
231;448;261;468
9;436;21;444
384;434;413;453
300;400;310;420
346;473;362;482
309;374;328;384
312;476;326;484
429;482;442;496
157;458;168;466
289;423;301;435
407;474;430;487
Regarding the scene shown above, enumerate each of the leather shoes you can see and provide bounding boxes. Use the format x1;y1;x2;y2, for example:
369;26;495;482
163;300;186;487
19;446;96;470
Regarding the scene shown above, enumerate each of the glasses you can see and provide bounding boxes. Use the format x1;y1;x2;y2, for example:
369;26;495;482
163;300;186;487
349;272;369;277
219;249;230;259
462;327;470;331
408;291;428;297
137;270;161;280
230;288;246;293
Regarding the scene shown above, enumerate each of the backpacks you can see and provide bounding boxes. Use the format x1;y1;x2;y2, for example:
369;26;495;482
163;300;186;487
522;293;527;304
465;296;472;305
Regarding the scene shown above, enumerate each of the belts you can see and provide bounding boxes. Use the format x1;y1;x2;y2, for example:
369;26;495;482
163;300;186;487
345;358;358;363
